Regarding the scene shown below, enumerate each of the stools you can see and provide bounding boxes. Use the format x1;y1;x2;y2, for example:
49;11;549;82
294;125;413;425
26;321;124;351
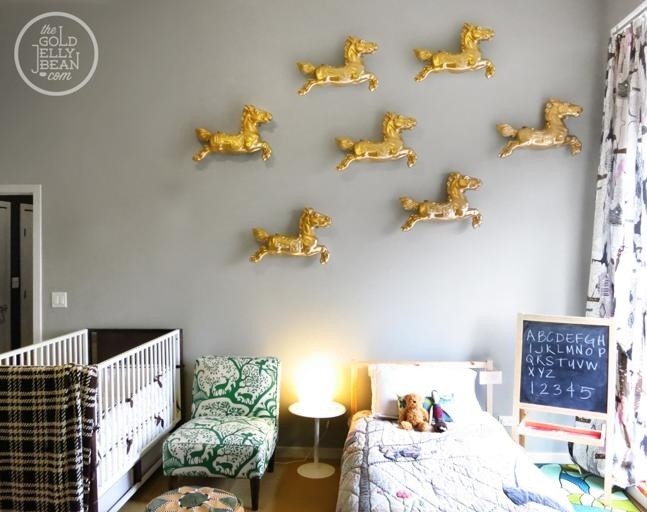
144;485;245;511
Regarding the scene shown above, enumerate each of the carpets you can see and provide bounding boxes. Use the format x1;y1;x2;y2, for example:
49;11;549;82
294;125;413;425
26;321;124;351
534;464;643;512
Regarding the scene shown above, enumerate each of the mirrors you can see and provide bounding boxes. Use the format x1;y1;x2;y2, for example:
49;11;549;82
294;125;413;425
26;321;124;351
0;184;42;351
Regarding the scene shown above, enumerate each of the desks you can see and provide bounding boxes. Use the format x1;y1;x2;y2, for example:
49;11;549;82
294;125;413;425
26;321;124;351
286;400;347;480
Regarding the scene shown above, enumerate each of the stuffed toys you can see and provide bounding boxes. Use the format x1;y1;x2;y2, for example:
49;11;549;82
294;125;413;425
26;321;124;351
425;390;453;432
398;391;428;432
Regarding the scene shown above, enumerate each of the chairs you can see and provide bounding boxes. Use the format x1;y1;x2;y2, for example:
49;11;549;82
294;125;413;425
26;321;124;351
161;355;281;511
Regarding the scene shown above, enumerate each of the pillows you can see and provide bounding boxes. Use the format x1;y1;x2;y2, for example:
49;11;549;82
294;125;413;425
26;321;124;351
368;362;481;417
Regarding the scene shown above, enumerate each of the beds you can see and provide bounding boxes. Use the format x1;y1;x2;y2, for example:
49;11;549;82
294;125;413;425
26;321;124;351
0;327;185;512
334;358;574;512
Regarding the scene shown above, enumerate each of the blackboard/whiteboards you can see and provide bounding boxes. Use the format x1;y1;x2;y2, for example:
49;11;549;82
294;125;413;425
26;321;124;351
511;311;616;419
